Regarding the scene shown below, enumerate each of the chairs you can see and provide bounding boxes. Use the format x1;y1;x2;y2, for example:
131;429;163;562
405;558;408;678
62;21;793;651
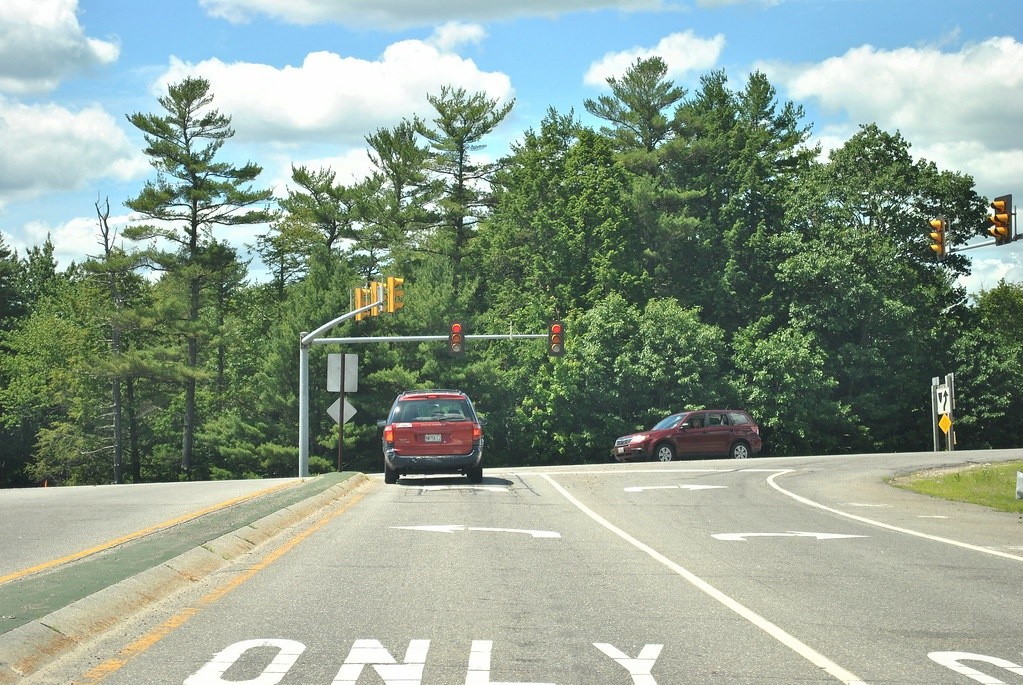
447;409;462;415
402;405;419;422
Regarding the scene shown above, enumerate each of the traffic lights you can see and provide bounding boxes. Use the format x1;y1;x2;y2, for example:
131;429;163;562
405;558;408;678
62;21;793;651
448;320;466;354
929;216;946;258
986;194;1013;246
369;281;388;317
385;276;405;313
354;288;370;322
547;320;565;358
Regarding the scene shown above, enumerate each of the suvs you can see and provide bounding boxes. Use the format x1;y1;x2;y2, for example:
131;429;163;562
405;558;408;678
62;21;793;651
612;407;763;463
375;387;490;484
